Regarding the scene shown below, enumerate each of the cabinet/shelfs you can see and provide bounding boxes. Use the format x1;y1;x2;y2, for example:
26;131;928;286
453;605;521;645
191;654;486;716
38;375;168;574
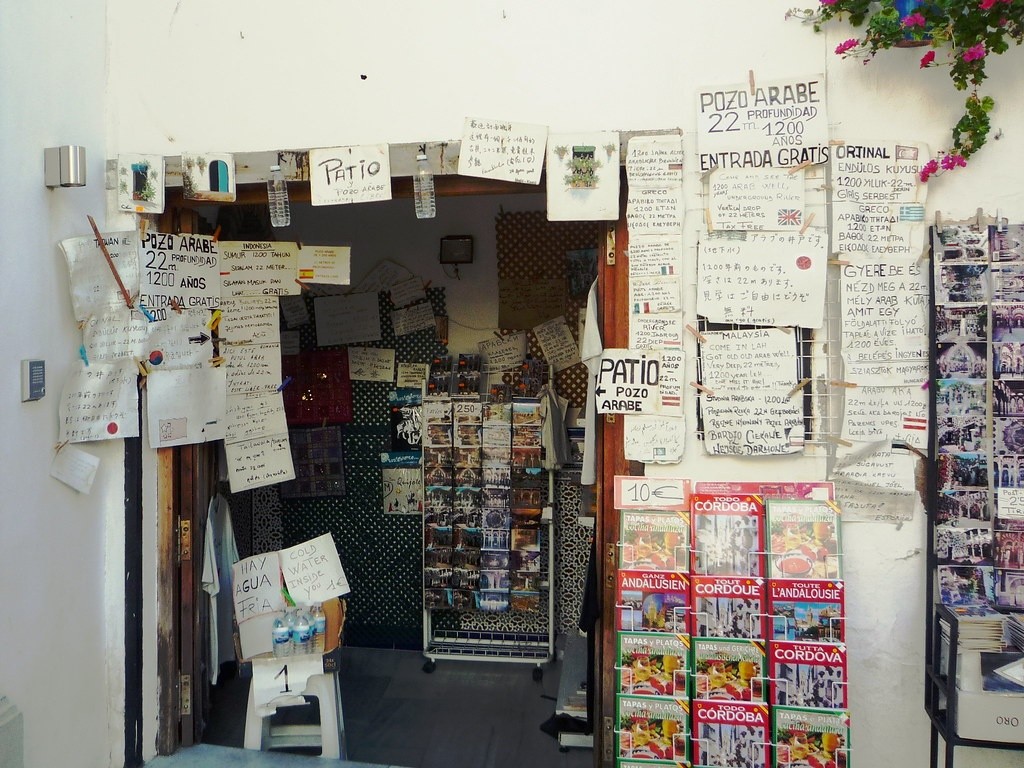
929;603;1023;768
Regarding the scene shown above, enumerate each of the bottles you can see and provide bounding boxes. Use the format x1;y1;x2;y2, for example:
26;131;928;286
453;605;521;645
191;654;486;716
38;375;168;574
267;166;291;227
272;601;325;655
413;155;436;218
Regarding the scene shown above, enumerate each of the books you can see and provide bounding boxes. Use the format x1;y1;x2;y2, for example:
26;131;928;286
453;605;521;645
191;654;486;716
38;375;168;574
616;493;851;768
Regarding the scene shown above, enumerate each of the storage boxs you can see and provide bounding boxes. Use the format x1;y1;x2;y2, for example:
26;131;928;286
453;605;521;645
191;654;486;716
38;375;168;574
954;683;1023;745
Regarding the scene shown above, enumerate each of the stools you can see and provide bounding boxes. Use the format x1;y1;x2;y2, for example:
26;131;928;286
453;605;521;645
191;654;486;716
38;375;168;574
242;647;347;759
